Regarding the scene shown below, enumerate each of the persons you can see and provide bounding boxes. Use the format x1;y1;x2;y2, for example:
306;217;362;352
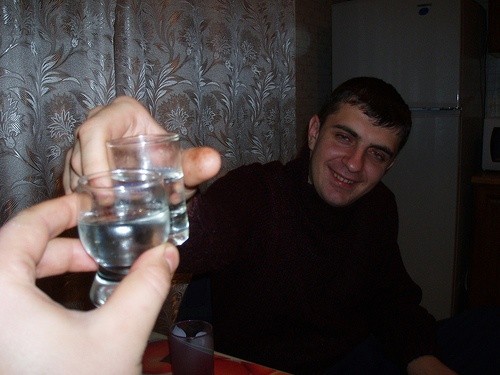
63;76;454;374
1;191;181;374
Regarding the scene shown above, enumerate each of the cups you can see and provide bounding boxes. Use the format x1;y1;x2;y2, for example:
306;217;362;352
105;131;189;247
75;168;172;310
167;318;215;375
